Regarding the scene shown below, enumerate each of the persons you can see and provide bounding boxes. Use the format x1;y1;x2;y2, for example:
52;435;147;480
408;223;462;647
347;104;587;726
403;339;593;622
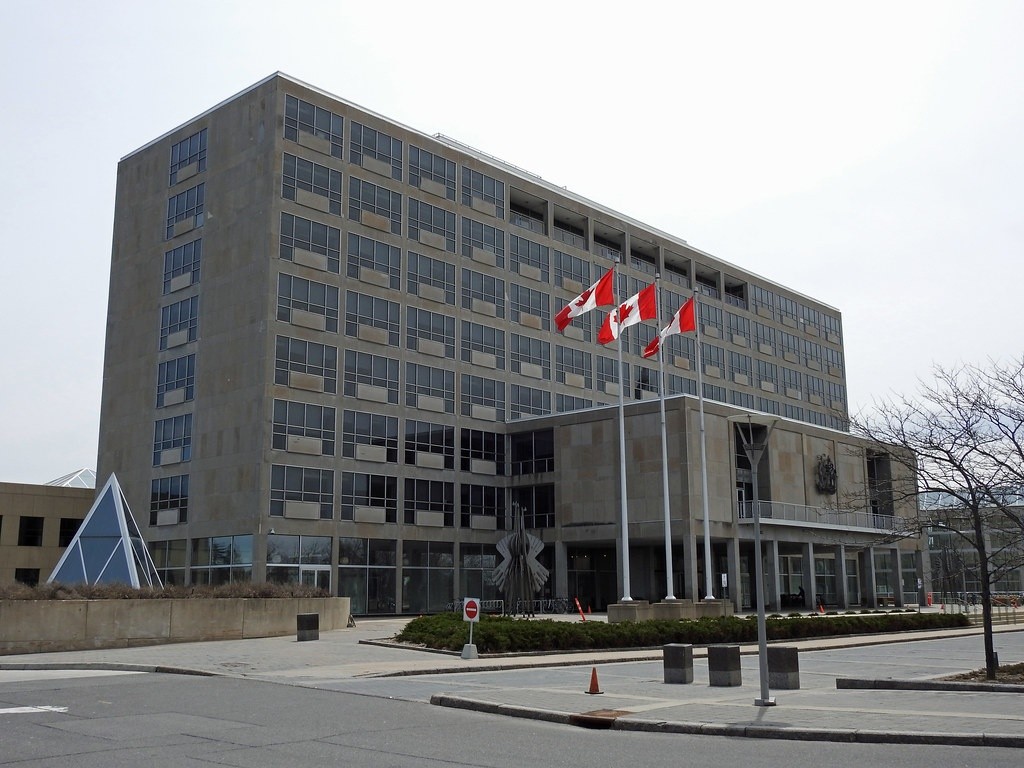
798;586;805;599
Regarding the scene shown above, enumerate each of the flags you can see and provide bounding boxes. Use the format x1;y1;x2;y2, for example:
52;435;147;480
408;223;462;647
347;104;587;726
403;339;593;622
553;267;614;332
643;296;696;359
598;281;657;345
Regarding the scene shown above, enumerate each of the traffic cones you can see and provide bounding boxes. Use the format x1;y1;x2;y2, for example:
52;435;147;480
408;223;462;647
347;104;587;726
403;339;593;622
588;606;592;615
819;605;825;613
585;667;605;694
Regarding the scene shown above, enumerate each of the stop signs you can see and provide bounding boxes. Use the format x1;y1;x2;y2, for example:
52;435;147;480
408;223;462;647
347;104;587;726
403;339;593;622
465;601;477;619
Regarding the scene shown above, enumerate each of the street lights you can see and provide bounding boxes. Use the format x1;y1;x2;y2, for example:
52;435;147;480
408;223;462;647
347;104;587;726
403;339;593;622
726;413;785;707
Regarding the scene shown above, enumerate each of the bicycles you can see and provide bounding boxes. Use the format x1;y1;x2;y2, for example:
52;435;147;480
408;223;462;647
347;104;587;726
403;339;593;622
376;594;396;611
445;598;464;612
543;597;575;614
